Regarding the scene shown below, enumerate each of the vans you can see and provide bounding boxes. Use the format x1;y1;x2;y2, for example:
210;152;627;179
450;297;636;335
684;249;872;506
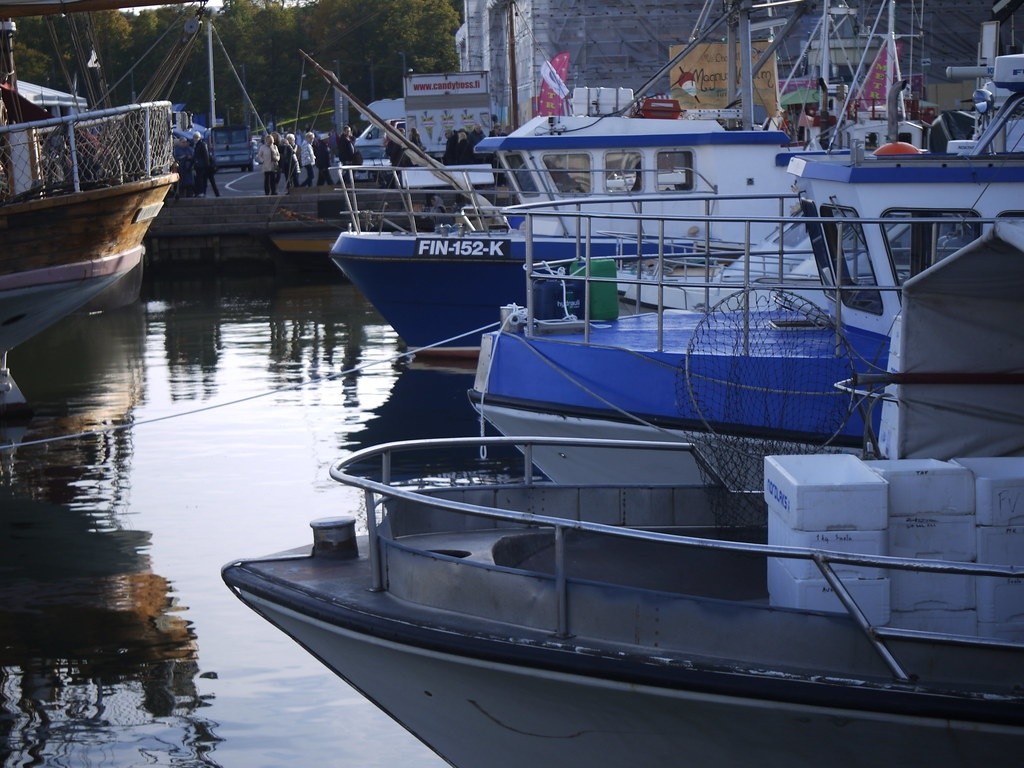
205;125;253;173
353;119;396;158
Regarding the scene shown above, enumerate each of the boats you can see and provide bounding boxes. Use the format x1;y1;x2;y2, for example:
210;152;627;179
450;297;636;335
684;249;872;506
0;0;1023;767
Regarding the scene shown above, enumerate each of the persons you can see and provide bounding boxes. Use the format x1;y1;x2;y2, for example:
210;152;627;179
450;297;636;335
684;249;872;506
430;196;451;233
443;122;488;191
488;122;507;187
256;123;364;195
172;131;220;198
386;128;425;188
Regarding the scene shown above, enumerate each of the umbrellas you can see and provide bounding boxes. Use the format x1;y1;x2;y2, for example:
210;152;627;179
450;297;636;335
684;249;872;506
780;87;832;142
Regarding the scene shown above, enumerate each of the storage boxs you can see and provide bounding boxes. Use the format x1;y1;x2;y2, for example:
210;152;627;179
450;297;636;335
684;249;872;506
764;454;1024;644
